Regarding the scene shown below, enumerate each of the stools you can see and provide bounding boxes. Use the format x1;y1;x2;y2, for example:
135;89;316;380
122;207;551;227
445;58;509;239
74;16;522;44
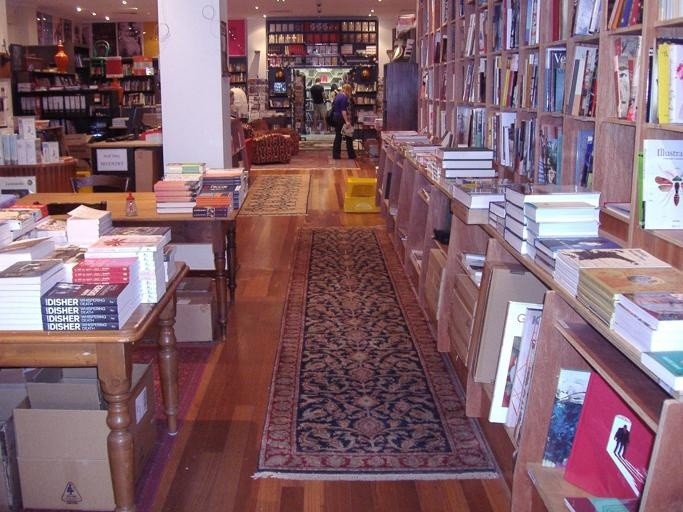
344;178;380;213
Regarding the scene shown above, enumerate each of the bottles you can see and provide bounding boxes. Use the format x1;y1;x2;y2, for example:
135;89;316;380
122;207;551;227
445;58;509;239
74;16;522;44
126;193;138;218
54;45;70;72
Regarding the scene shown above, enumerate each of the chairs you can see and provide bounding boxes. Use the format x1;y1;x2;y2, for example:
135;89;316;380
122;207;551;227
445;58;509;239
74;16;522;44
242;120;300;164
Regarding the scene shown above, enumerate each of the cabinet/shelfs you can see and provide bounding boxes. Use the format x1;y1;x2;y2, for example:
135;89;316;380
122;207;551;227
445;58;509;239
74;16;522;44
375;0;683;512
229;56;249;111
266;16;378;140
0;44;162;193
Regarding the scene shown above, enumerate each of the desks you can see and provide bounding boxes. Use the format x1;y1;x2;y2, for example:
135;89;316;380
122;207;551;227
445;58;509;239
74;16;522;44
1;260;192;512
1;191;246;342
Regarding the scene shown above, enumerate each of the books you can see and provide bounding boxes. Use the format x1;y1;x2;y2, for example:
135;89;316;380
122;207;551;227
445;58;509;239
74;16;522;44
251;19;292;129
154;163;249;217
291;21;378;68
0;195;176;333
351;82;379;157
1;44;157;164
378;1;682;512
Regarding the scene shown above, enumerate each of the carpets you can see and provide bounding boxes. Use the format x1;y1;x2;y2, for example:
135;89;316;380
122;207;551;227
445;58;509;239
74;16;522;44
133;343;223;512
253;226;503;479
249;134;366;170
238;174;311;215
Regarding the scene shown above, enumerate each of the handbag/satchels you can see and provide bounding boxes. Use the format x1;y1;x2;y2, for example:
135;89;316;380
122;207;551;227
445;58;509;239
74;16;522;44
341;122;354;137
325;108;336;128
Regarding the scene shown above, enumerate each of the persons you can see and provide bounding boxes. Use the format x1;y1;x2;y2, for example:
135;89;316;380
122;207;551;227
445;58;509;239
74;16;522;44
308;80;329;133
614;424;630;456
330;83;358;158
325;84;338;103
